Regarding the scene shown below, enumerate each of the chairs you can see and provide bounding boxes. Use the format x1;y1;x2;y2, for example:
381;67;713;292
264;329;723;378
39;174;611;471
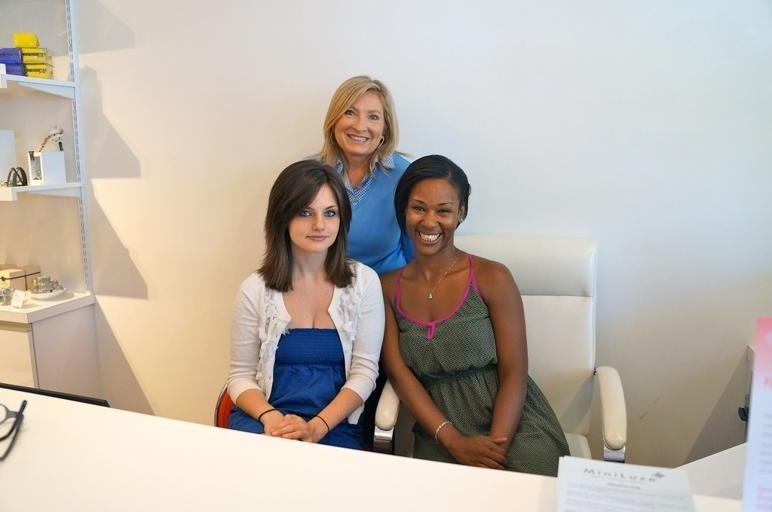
212;380;237;428
0;382;113;407
371;229;630;469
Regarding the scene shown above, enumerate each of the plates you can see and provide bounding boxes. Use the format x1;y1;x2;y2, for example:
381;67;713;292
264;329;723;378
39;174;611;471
27;288;66;300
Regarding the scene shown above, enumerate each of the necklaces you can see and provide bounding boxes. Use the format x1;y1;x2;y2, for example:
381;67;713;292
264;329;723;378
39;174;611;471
427;260;456;300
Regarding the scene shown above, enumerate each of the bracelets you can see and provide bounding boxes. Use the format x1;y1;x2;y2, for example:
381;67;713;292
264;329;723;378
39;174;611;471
258;409;278;422
315;415;329;431
435;421;452;441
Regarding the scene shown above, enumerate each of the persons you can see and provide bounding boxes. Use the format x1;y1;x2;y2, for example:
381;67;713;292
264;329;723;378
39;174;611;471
227;160;386;450
304;76;416;275
380;155;569;477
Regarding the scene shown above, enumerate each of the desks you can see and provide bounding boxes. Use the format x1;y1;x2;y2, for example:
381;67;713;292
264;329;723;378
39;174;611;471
1;384;719;512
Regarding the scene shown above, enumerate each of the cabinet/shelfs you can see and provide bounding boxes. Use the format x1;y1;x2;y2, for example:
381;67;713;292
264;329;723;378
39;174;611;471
0;0;97;389
